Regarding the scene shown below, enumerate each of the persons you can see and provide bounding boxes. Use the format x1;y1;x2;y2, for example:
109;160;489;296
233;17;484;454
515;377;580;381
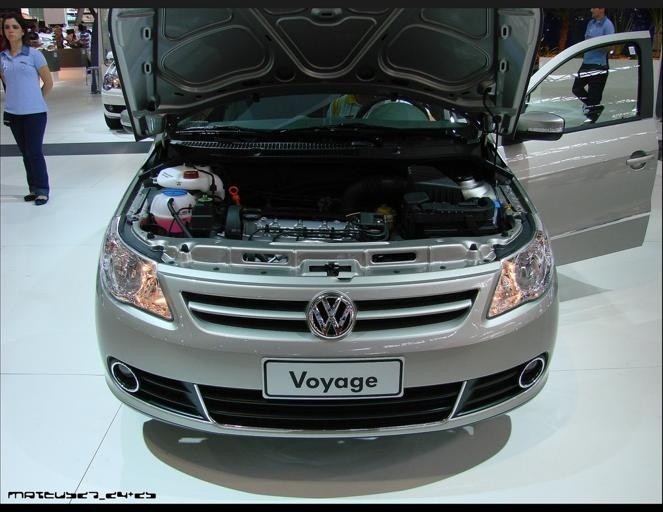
1;15;11;126
572;6;616;125
22;20;92;71
1;11;54;206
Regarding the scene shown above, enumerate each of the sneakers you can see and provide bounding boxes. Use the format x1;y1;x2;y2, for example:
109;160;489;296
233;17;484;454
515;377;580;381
584;104;605;124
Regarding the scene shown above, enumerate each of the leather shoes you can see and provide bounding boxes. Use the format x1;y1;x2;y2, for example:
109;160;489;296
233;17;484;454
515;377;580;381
23;193;37;201
35;194;49;205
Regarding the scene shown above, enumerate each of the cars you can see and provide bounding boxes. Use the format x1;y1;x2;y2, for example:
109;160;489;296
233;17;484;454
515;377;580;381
66;11;94;24
